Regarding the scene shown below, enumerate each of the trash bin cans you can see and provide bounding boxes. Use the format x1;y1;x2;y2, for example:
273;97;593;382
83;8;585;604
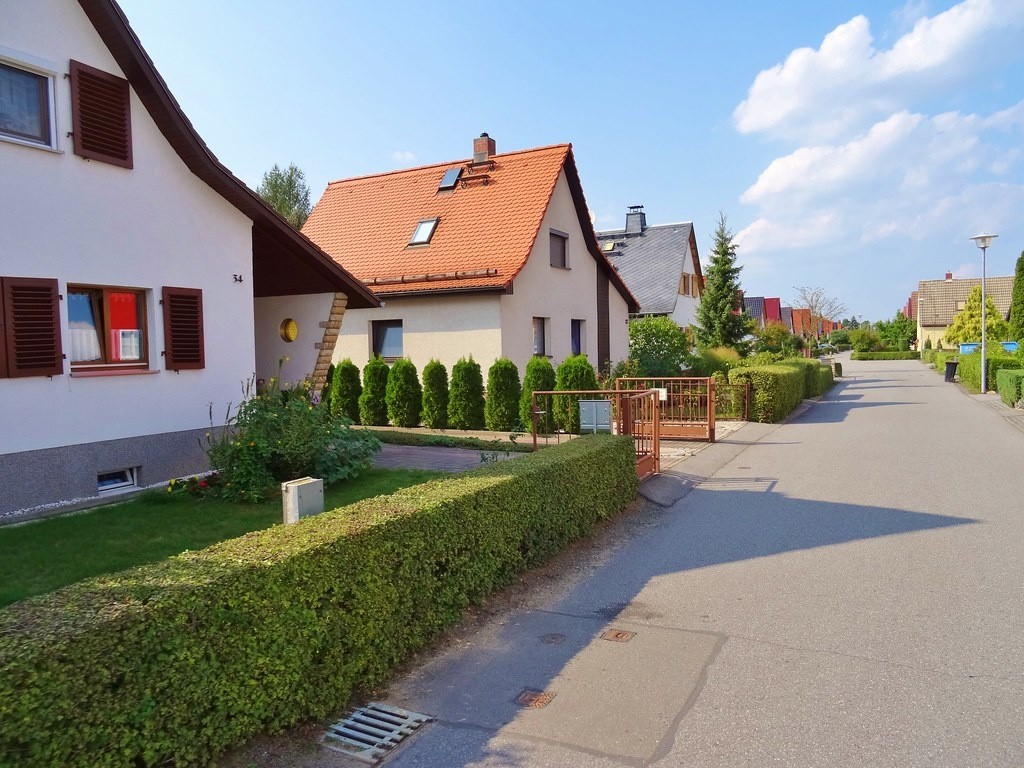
943;361;959;383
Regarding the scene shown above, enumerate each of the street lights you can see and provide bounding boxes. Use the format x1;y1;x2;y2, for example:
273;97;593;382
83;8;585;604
918;297;925;360
968;231;1000;395
